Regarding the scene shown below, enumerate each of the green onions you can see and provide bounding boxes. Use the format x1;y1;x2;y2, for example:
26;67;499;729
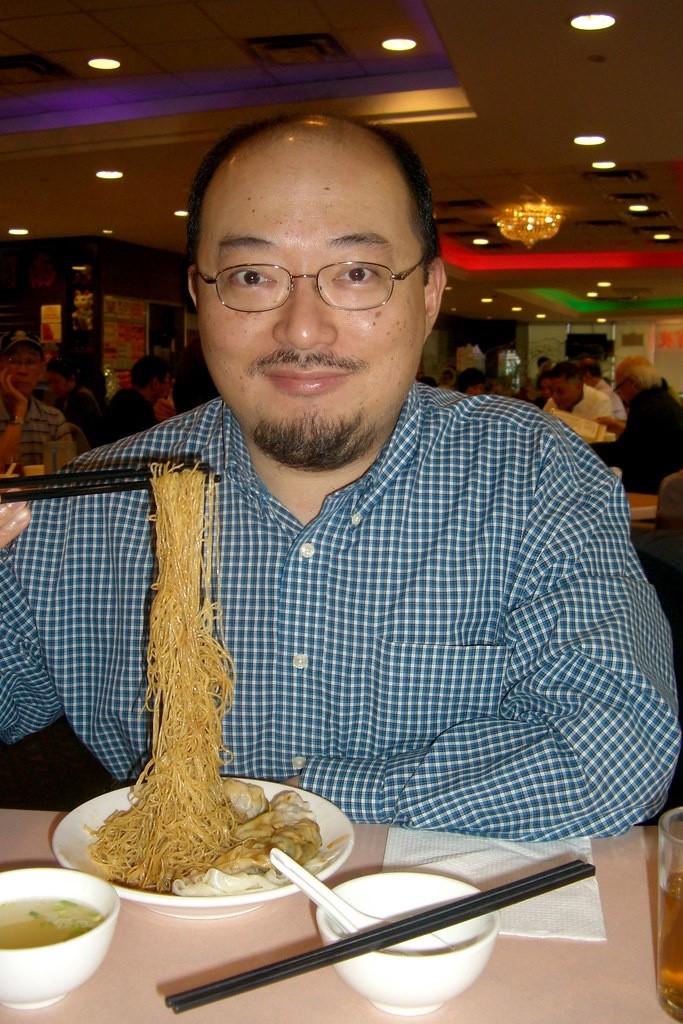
29;899;106;940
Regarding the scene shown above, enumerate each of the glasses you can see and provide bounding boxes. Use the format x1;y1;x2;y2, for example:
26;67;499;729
6;357;38;367
162;379;175;389
612;375;629;396
197;259;423;310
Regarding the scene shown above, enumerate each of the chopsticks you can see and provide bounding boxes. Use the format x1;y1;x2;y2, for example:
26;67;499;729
0;462;222;504
164;858;597;1014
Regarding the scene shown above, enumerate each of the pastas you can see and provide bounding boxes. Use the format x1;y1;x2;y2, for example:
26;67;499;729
82;459;250;895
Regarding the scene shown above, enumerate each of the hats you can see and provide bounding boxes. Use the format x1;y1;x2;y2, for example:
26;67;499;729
0;329;44;363
47;357;80;381
537;357;550;371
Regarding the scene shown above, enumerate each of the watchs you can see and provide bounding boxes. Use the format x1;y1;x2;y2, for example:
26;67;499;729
10;416;24;425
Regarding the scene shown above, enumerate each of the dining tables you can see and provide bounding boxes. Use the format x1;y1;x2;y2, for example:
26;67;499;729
0;805;683;1024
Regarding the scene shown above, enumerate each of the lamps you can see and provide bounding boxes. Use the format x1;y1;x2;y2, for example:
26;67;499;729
493;198;566;249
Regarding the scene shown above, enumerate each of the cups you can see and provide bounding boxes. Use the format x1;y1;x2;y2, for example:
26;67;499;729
655;806;683;1024
43;440;77;474
4;451;20;474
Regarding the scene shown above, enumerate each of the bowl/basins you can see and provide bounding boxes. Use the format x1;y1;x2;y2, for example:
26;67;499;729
23;465;45;476
0;866;121;1010
316;871;502;1018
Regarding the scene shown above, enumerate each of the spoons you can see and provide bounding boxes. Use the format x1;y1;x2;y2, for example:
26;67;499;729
269;846;454;955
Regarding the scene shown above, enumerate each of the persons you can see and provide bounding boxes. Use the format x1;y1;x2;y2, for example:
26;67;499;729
0;331;72;466
531;356;552;387
541;362;616;442
416;367;486;395
579;358;627;420
46;356;102;438
532;370;554;410
0;112;683;842
589;355;683;495
94;355;172;448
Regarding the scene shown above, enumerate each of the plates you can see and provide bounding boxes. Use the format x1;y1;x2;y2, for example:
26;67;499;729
52;776;355;919
